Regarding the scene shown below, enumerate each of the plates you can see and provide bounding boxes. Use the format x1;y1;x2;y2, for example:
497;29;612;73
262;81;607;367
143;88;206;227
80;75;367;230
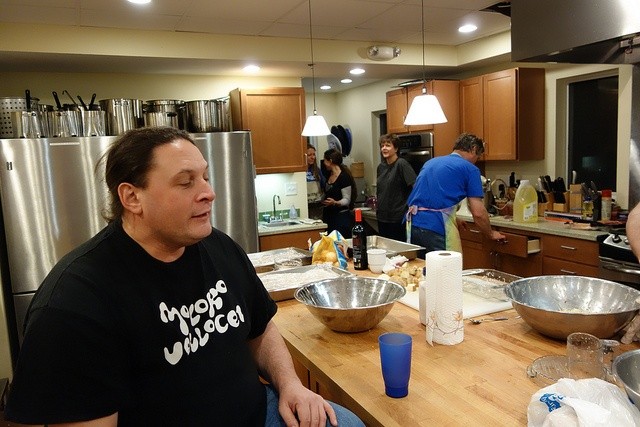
526;354;608;390
271;255;304;272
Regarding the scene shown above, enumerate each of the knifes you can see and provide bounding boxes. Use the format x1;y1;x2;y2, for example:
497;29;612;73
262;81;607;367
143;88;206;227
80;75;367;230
537;176;566;203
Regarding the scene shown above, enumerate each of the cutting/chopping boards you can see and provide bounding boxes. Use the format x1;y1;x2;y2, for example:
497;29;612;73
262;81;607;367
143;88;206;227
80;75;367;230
398;284;515;320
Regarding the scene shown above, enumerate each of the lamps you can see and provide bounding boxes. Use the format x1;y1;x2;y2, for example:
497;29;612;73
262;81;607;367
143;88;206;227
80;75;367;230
300;1;333;136
402;0;449;127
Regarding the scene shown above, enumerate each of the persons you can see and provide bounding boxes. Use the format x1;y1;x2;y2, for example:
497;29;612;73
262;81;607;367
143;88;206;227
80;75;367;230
307;144;326;220
377;134;416;242
323;149;357;239
405;132;507;260
1;127;364;426
625;201;640;262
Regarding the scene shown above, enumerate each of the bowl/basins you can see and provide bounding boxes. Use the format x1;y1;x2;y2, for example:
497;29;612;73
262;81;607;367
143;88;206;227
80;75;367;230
294;275;406;333
502;275;640;342
613;348;640;410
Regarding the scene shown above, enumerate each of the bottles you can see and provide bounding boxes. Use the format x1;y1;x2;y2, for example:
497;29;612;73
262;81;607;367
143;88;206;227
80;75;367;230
581;195;593;222
352;206;367;272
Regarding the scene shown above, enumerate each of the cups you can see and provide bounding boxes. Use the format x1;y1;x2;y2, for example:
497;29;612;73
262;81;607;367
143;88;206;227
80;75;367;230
597;338;620;378
377;332;414;398
565;332;604;378
366;247;387;273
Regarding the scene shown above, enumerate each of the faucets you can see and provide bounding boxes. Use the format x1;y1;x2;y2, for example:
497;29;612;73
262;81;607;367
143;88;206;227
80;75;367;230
273;194;281;219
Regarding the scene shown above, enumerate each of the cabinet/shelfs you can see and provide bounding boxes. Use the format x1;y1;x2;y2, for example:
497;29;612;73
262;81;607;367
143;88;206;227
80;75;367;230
457;219;611;278
386;79;460;157
460;66;545;161
256;217;328;252
230;88;309;175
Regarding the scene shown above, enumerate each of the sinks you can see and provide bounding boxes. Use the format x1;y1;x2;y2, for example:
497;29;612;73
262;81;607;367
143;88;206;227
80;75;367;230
258;219;304;229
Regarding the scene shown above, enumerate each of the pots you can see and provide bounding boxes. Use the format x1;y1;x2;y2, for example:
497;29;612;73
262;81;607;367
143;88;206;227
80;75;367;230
143;97;182;131
10;110;42;140
100;99;140;134
58;85;106;138
1;89;39;139
43;89;79;139
183;96;232;131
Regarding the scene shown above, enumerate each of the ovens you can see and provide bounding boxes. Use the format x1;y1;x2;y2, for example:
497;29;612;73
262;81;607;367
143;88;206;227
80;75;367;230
599;232;640;291
395;131;434;175
